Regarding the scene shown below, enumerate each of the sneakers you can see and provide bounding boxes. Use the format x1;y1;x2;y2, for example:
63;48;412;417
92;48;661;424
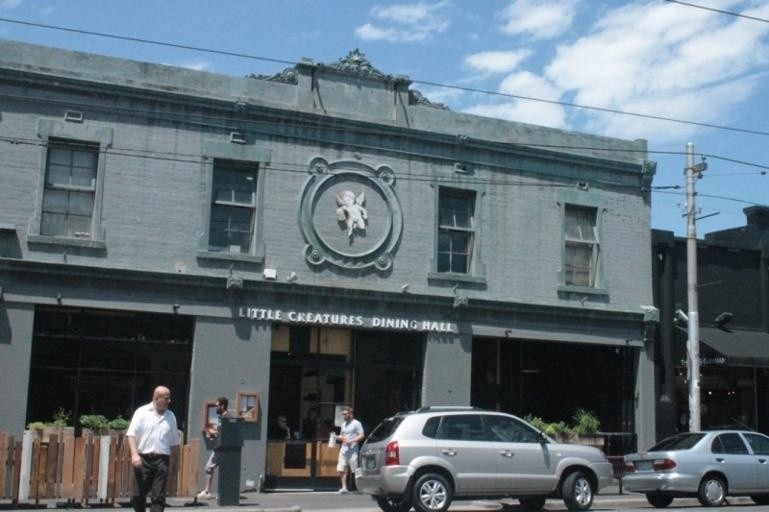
337;489;348;494
197;491;214;500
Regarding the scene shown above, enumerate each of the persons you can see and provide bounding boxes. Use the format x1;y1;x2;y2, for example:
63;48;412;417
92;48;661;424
303;407;329;438
333;407;365;495
196;396;235;500
337;190;368;237
268;414;291;440
126;385;181;512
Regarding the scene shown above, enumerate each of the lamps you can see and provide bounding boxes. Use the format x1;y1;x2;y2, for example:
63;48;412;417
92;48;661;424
286;270;298;281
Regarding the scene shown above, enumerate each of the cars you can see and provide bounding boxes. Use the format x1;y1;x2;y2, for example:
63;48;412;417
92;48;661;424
622;429;768;508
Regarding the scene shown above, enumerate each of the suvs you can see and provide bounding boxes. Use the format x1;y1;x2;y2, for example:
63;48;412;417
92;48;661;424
356;405;615;511
